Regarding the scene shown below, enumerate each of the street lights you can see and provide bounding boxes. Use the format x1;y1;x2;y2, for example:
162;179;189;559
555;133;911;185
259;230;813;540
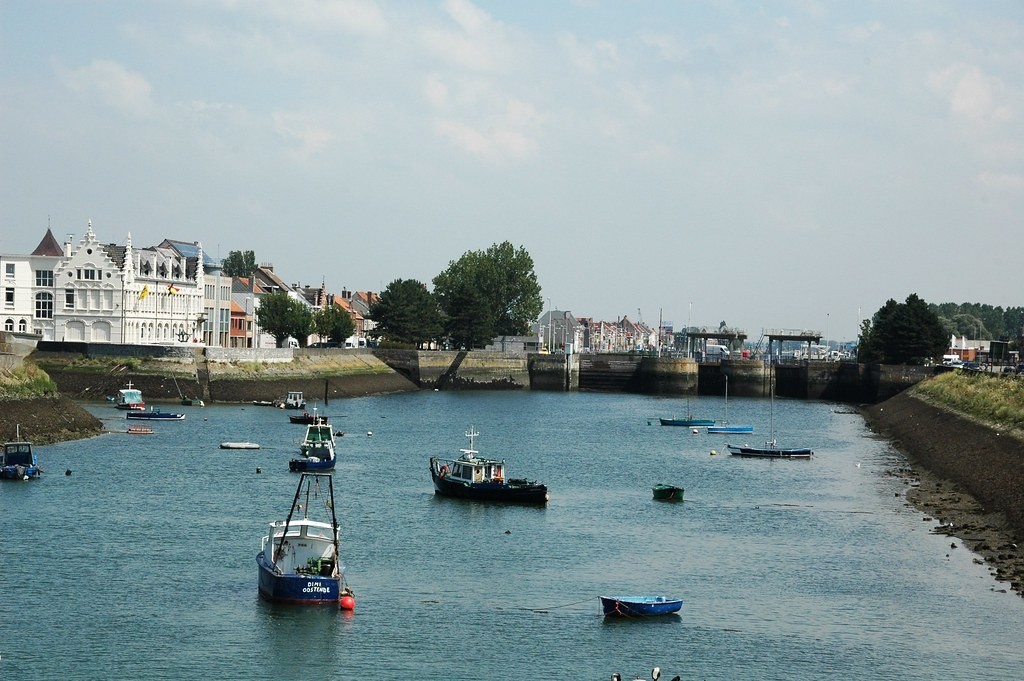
245;297;252;347
547;296;552;353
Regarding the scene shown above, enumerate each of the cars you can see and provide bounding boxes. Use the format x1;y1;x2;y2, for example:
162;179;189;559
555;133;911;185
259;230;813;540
951;361;963;368
1004;366;1016;373
1015;363;1024;374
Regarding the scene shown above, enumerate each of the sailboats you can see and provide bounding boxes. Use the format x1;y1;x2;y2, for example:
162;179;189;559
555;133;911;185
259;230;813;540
726;384;814;459
707;374;754;434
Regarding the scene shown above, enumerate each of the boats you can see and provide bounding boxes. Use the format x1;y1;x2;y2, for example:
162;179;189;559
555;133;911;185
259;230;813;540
287;411;329;425
255;474;357;609
181;395;204;408
651;483;685;499
658;399;716;426
0;440;44;481
288;439;338;472
429;425;506;499
126;422;155;435
480;477;550;504
219;441;261;450
596;594;684;617
254;389;307;410
298;417;337;452
125;406;187;421
115;382;147;411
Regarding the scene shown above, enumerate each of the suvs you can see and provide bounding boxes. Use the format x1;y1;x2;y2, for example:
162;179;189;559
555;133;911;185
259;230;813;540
962;362;983;371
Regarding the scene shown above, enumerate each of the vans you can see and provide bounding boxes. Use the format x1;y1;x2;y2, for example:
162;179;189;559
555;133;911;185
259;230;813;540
703;345;730;355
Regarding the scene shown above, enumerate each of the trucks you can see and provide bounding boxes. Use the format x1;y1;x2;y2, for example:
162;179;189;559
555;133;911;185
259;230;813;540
943;357;954;365
827;350;845;361
